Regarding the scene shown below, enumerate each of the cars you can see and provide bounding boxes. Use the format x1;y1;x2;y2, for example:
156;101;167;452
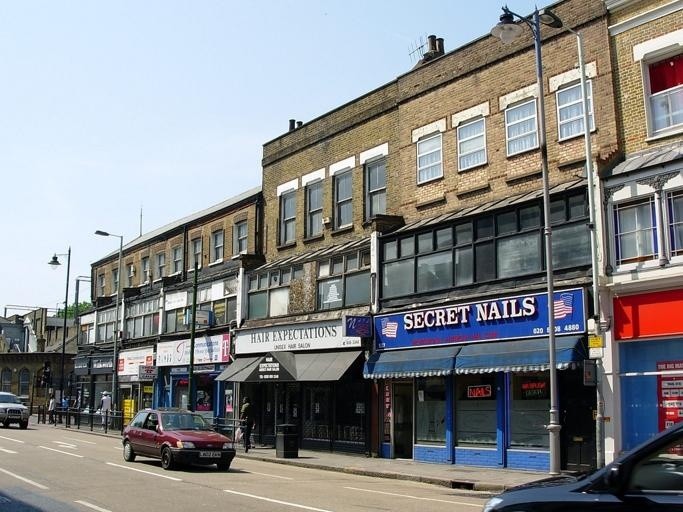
481;420;682;512
121;407;235;471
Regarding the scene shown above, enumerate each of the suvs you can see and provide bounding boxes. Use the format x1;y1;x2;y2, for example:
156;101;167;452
0;391;29;429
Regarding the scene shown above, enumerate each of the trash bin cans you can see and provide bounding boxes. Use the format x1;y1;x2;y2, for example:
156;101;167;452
275;423;300;458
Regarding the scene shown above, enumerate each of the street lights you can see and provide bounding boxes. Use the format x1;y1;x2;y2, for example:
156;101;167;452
47;245;71;424
538;6;607;475
488;4;564;477
95;230;123;414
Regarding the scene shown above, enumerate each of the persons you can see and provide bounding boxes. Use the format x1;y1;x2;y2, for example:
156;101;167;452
97;391;111;431
240;396;254;448
47;393;56;424
62;399;69;418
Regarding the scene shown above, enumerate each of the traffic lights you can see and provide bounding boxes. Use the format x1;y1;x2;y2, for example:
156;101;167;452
41;369;50;388
